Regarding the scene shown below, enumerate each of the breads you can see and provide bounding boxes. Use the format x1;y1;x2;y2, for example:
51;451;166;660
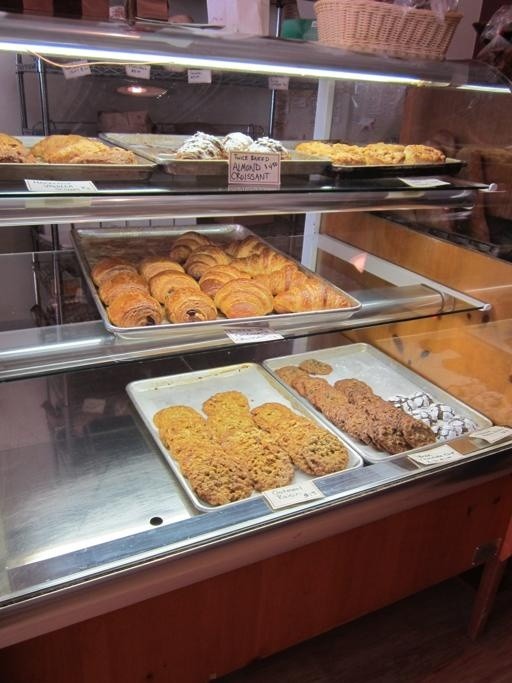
175;130;293;162
80;232;353;329
295;140;445;168
1;132;140;166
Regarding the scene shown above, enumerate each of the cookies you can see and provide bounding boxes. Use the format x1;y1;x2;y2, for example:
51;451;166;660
272;359;438;453
153;390;349;505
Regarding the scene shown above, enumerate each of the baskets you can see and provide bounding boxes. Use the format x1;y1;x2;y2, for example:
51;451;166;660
313;0;464;62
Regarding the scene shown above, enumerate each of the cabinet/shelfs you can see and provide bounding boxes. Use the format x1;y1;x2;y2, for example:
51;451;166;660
2;8;512;683
11;49;286;460
